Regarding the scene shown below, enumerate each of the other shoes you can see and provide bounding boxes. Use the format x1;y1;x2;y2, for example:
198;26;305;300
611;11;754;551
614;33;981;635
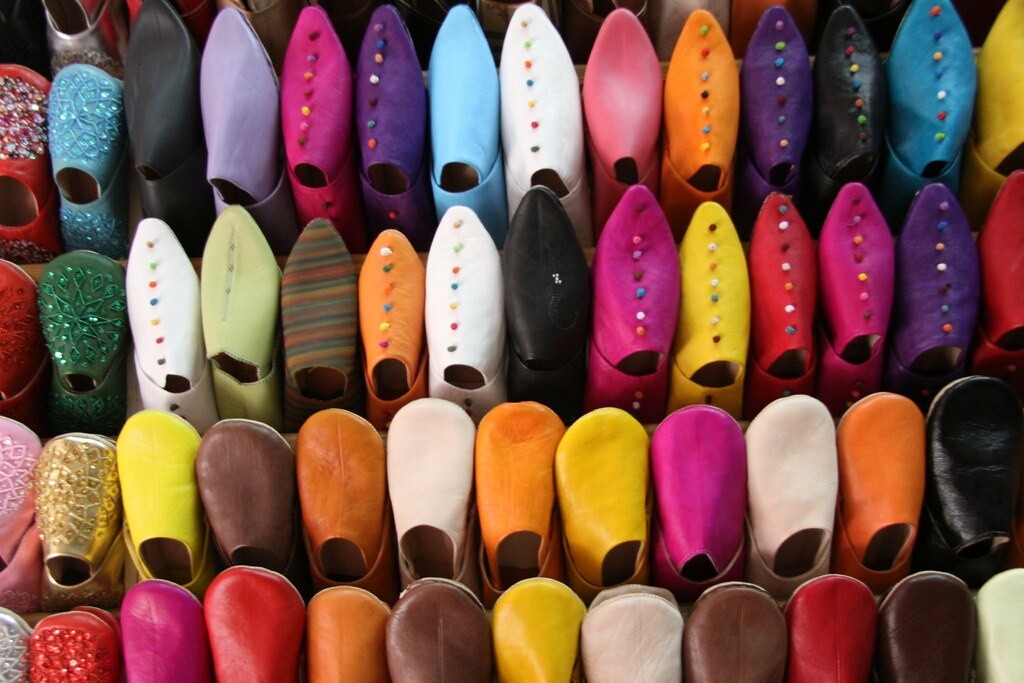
0;0;1024;683
